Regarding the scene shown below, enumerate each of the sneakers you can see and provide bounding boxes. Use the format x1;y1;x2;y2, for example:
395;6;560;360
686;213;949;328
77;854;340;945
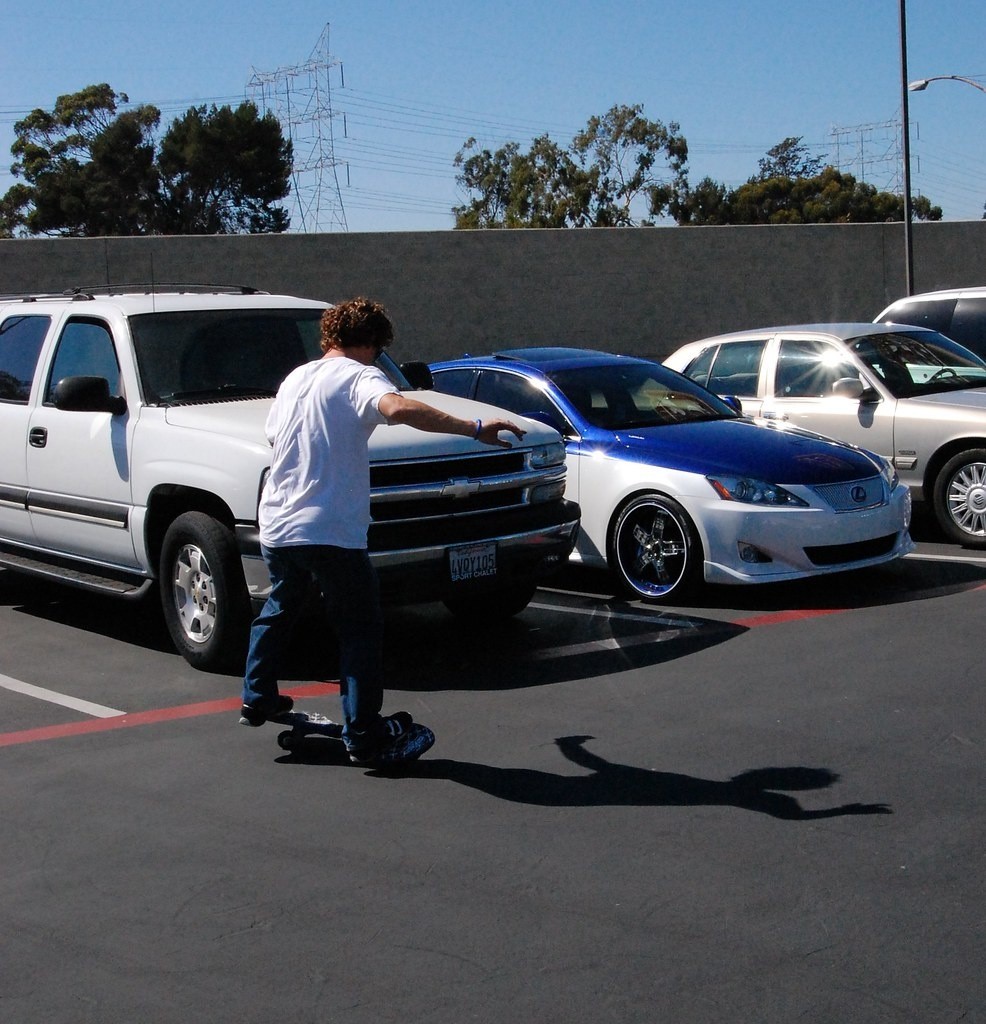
240;694;292;727
349;710;413;762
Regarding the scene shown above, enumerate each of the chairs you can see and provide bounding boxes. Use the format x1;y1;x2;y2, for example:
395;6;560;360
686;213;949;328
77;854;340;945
564;378;593;412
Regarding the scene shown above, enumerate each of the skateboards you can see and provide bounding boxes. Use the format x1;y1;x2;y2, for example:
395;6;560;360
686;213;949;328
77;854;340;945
266;710;436;774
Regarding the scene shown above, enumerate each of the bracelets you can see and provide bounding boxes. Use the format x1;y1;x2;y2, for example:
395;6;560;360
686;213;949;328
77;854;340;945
474;419;481;440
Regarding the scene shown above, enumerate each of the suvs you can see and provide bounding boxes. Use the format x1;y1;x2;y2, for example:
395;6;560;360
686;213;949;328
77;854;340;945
0;283;584;680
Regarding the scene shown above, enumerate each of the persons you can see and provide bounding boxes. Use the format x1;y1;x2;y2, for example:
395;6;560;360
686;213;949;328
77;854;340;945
241;295;528;766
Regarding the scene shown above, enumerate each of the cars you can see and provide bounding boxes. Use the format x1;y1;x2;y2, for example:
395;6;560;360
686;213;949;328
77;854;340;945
399;346;918;604
629;322;985;548
853;288;985;381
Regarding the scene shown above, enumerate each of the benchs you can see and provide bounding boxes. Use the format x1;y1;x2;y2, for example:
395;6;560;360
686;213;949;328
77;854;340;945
696;371;758;394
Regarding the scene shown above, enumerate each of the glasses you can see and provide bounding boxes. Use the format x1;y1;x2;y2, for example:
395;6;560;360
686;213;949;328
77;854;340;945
375;348;386;358
